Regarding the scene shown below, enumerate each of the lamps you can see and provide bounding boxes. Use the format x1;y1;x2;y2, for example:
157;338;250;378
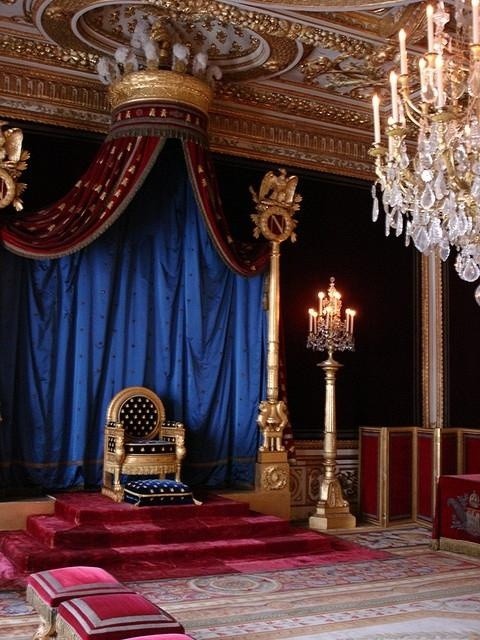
367;2;480;305
306;276;357;529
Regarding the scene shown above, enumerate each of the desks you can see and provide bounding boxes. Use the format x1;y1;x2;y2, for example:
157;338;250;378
432;475;480;559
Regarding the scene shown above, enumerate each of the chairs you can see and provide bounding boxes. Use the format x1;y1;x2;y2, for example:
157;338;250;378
103;386;188;501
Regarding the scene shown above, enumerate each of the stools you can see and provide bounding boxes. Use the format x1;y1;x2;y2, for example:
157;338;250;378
23;566;196;640
122;479;191;506
